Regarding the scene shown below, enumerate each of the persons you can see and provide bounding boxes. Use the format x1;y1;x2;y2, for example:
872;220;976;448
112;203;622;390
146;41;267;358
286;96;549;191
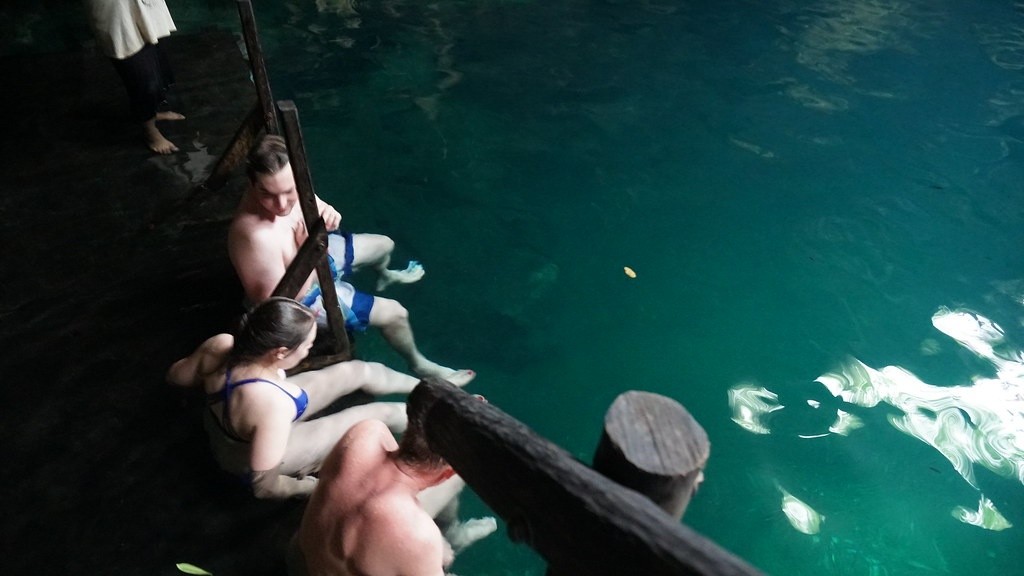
82;0;186;154
227;135;342;316
299;419;467;576
180;296;421;471
